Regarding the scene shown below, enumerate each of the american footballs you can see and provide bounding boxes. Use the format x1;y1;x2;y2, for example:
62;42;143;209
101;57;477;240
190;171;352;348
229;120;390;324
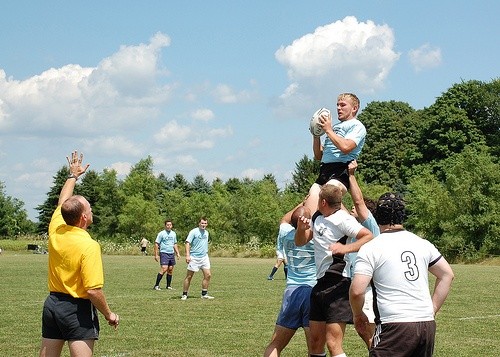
309;107;332;137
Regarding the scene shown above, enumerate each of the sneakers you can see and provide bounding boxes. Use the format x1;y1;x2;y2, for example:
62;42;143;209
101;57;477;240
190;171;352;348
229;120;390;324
201;294;214;299
268;276;276;280
180;295;187;300
166;286;176;290
154;286;161;291
